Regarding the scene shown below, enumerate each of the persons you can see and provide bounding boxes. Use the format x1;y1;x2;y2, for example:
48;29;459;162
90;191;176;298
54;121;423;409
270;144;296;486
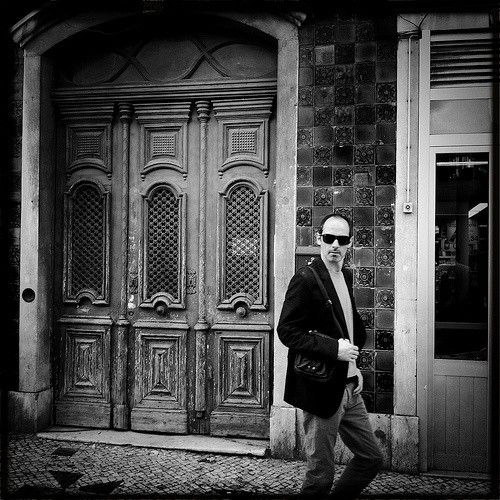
277;213;385;500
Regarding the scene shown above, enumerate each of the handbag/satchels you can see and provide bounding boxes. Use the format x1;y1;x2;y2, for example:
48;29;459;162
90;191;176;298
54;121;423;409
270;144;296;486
294;330;338;383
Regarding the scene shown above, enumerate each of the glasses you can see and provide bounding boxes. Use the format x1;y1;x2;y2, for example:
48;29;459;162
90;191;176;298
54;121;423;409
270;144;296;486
318;233;351;245
